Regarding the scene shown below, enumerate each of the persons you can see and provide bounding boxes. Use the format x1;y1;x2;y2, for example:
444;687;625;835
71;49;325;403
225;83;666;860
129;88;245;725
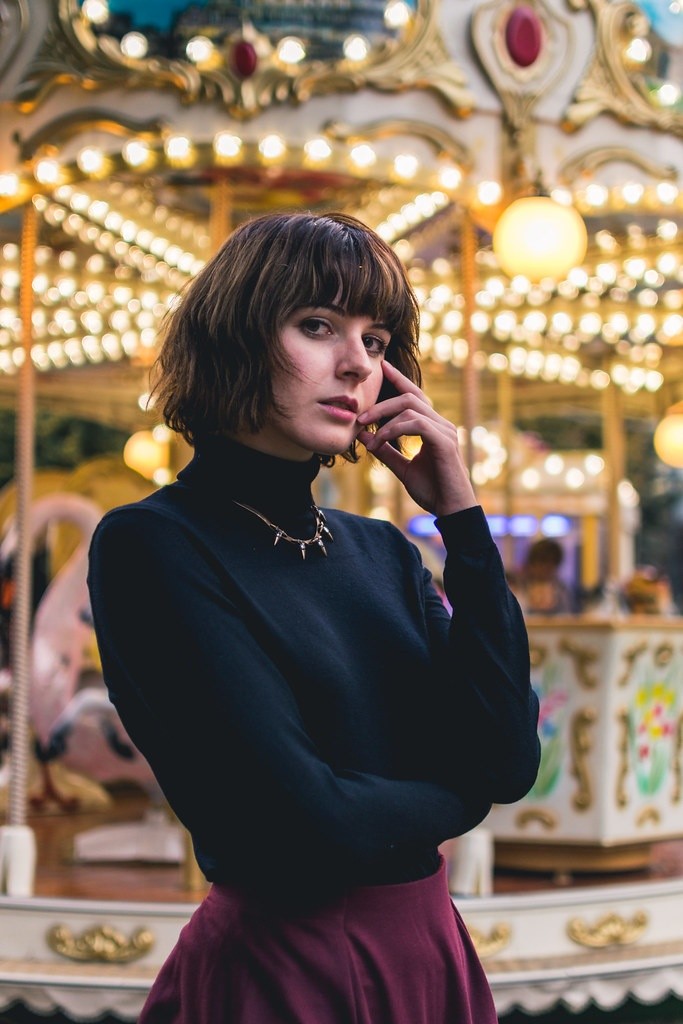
502;535;576;617
85;209;542;1024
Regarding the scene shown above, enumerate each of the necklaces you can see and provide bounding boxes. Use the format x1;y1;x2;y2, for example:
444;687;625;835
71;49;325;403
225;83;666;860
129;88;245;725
228;497;335;562
